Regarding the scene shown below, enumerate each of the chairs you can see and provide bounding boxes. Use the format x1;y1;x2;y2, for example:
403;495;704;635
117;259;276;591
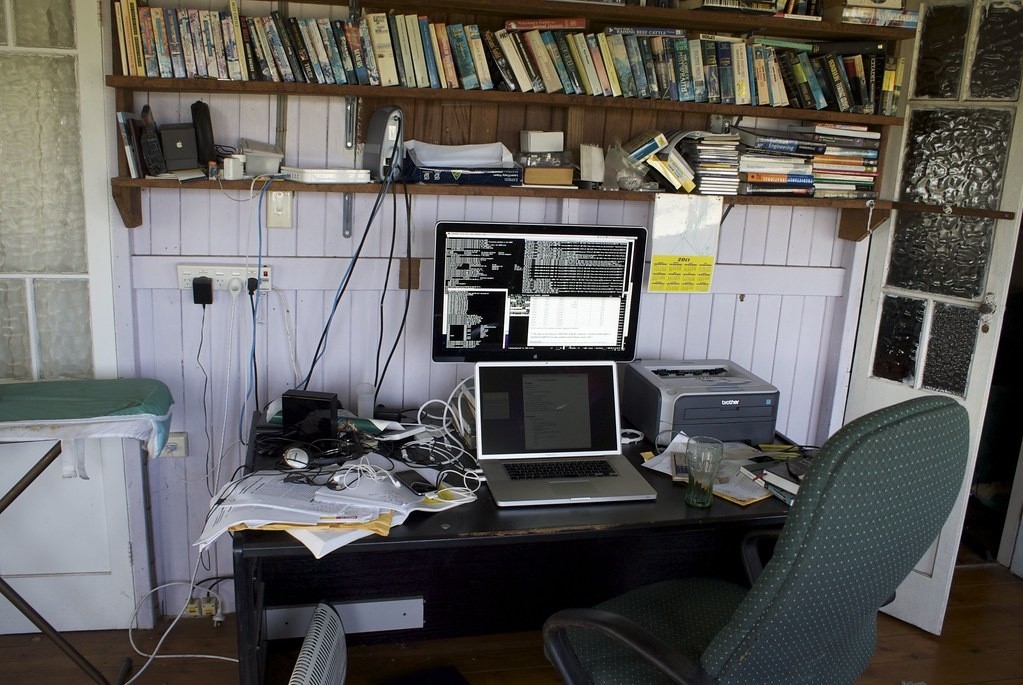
539;394;975;684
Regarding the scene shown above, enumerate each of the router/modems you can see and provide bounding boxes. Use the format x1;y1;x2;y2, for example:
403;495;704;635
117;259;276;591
361;104;407;183
280;165;370;184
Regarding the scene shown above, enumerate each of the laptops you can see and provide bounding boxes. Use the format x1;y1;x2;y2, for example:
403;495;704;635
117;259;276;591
474;360;657;508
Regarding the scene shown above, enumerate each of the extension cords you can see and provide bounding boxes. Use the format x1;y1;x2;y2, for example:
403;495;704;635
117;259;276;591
176;263;272;292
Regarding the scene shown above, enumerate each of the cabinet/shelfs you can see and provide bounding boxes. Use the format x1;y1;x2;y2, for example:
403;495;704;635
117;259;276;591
105;0;919;247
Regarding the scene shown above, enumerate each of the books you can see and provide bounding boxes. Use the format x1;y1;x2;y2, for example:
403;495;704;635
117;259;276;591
761;447;820;496
192;451;476;560
114;1;923;119
738;459;797;508
658;118;883;201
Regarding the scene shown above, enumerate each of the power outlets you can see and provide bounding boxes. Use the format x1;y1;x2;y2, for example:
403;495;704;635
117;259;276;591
176;265;273;295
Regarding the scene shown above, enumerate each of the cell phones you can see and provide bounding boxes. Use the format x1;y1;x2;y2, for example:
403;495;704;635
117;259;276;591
394;470;438;496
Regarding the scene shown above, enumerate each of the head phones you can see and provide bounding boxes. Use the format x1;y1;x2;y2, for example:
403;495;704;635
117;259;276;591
283;446;313;470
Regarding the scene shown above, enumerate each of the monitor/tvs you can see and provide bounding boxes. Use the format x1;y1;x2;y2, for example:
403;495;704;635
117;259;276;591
431;220;648;364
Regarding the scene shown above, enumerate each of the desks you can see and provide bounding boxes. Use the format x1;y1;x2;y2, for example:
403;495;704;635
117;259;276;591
233;413;828;685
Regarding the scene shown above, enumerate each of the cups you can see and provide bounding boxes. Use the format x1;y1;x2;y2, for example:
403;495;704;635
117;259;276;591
682;435;724;507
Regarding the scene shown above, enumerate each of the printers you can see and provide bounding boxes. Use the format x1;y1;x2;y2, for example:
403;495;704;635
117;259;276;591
621;358;780;447
